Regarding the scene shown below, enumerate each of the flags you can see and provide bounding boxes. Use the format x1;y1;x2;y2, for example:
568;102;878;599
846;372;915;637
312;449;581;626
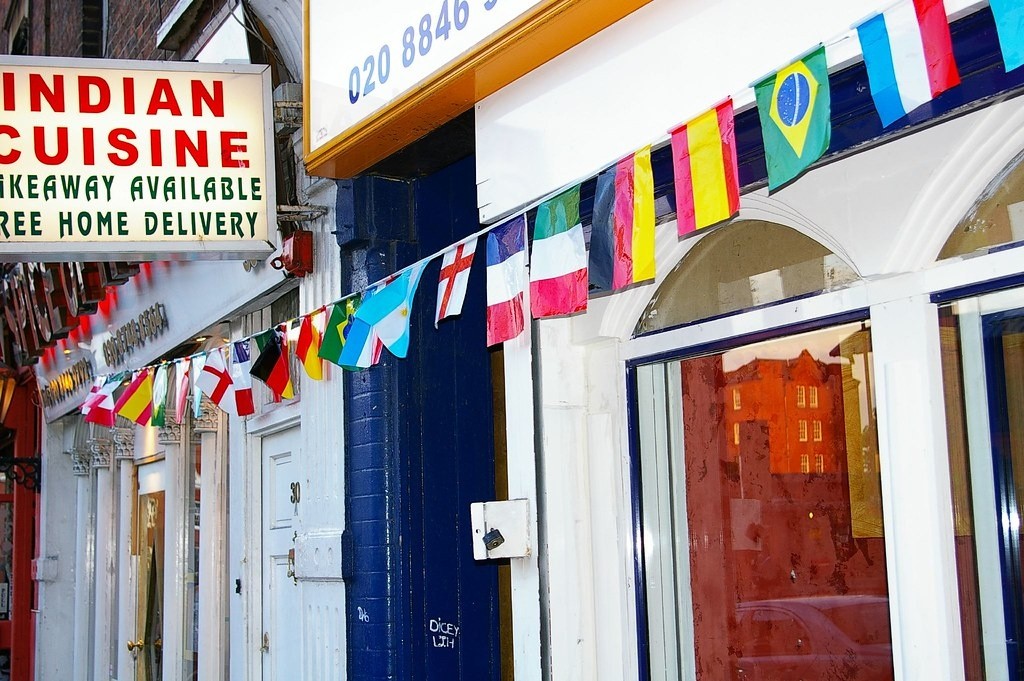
317;277;394;372
353;260;430;358
111;352;206;429
530;183;587;319
194;325;295;417
434;236;478;329
988;0;1024;73
295;306;326;380
671;98;740;238
78;370;129;427
587;143;657;294
856;0;961;129
754;45;831;191
486;213;525;347
